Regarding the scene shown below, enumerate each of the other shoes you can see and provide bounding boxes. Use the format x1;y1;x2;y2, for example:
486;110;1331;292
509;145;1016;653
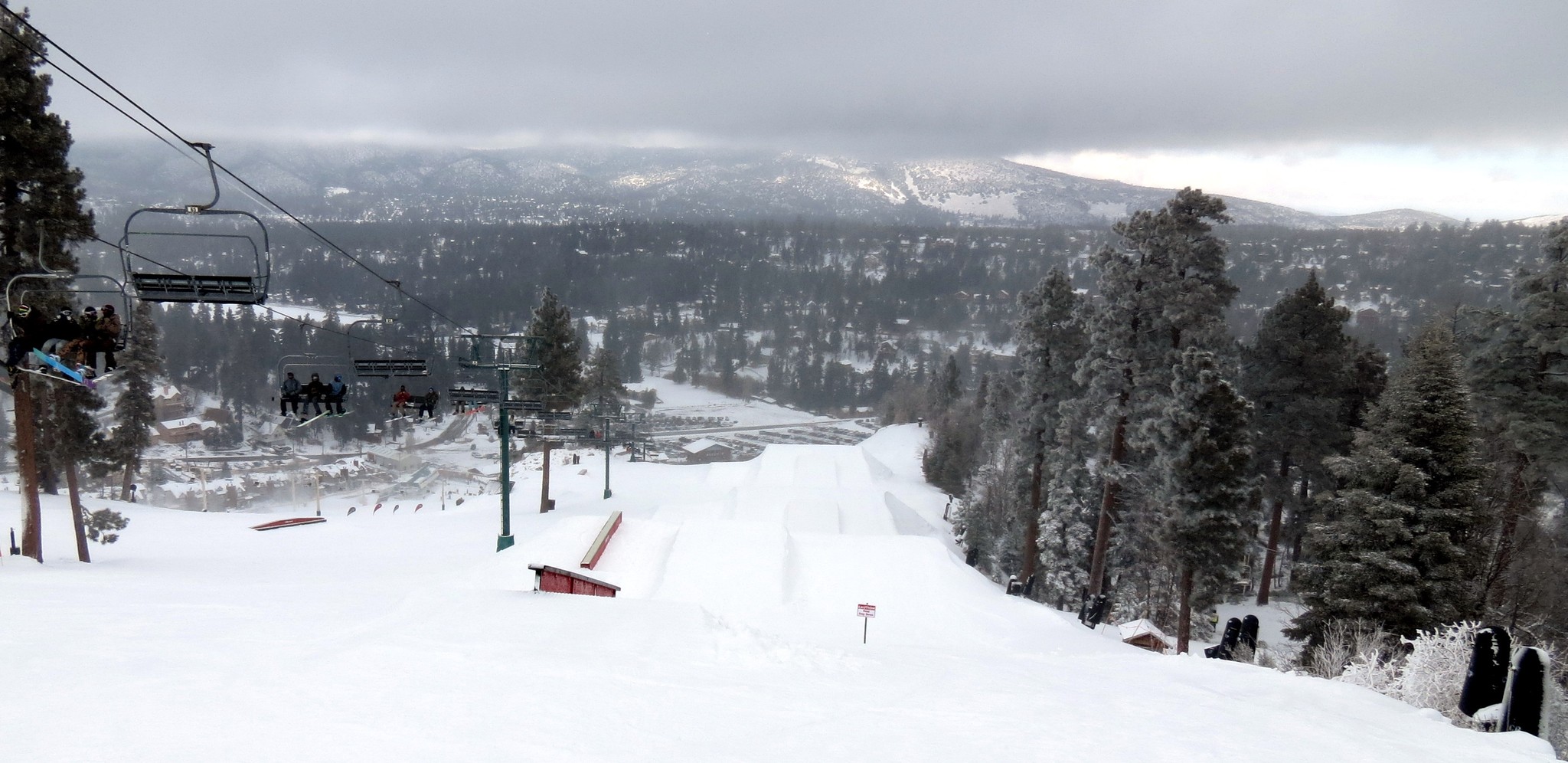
402;413;406;417
419;416;422;418
456;410;478;413
6;356;21;367
104;366;118;373
281;412;286;416
85;371;97;380
303;411;308;414
337;409;342;414
326;411;333;415
315;412;322;416
391;416;397;419
294;410;296;413
35;354;83;374
428;417;433;419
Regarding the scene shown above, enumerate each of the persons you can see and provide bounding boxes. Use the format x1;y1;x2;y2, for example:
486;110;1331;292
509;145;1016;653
35;306;78;373
508;415;516;437
452;386;466;415
49;306;100;370
498;418;500;438
467;388;478;412
390;385;410;417
300;373;324;417
324;372;347;415
85;305;121;378
0;304;49;377
280;372;302;416
415;387;439;419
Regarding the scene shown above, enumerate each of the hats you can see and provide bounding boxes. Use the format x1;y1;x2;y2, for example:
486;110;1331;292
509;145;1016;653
60;307;73;317
334;371;342;377
101;305;114;315
84;306;96;315
19;304;31;314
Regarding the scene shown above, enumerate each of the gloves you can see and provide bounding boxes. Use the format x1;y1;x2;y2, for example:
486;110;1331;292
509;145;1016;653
7;311;14;319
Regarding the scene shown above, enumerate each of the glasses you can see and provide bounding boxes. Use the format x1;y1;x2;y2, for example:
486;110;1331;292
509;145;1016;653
63;311;73;316
102;305;106;310
90;311;98;316
18;313;28;317
